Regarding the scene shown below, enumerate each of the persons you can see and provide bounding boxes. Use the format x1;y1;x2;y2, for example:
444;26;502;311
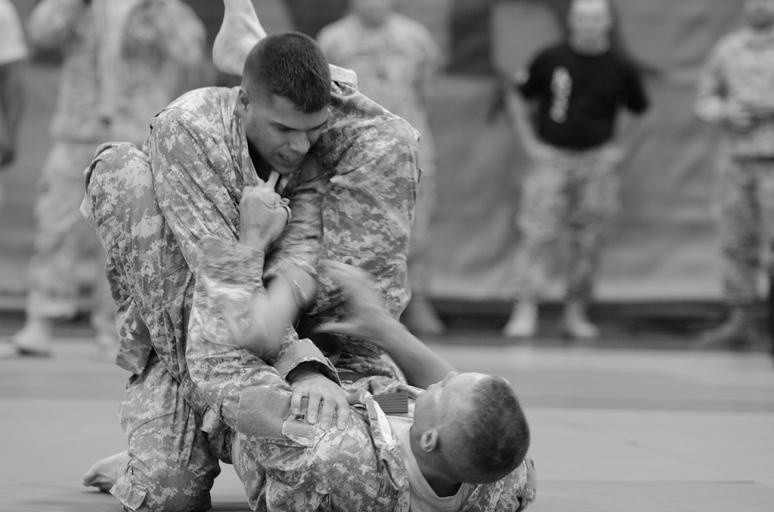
81;0;537;511
80;32;366;511
0;0;772;356
